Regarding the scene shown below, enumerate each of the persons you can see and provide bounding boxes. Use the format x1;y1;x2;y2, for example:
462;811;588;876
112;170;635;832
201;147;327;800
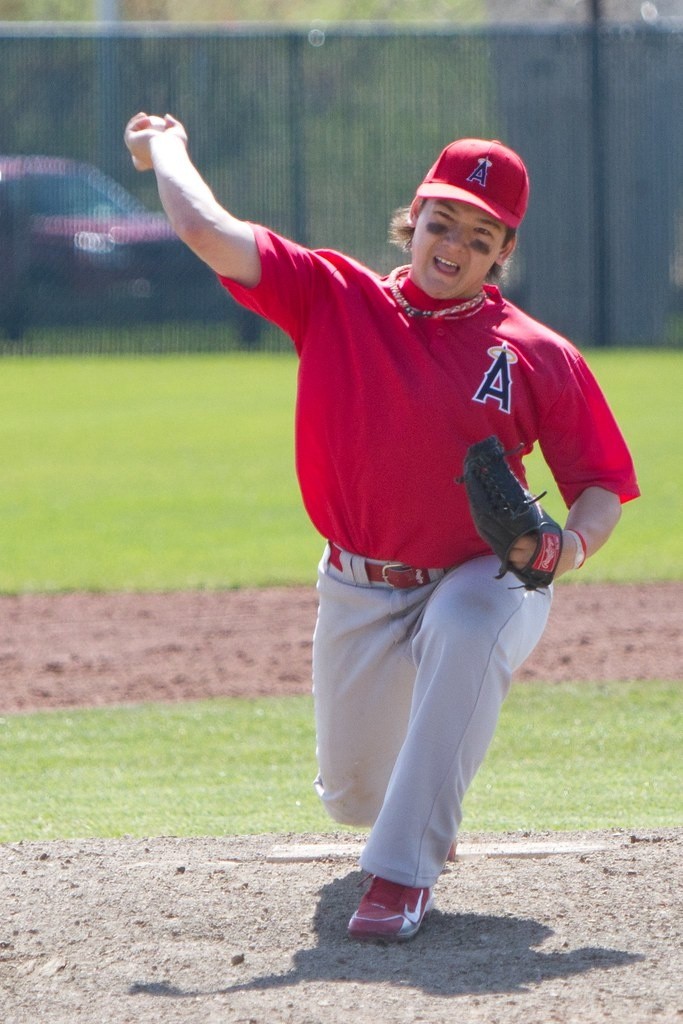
124;111;643;940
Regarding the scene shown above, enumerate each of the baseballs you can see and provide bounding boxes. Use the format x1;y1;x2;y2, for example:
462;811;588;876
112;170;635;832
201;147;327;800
144;114;166;132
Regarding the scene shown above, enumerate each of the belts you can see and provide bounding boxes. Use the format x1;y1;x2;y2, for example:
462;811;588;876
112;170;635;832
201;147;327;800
328;543;452;590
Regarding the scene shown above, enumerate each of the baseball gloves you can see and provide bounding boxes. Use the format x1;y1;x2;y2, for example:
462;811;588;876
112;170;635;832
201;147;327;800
454;439;563;599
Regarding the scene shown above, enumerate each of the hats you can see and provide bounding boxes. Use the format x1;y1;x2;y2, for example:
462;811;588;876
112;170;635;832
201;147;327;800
417;138;529;228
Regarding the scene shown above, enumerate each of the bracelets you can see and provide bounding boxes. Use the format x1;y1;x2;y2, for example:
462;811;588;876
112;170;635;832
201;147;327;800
565;528;586;570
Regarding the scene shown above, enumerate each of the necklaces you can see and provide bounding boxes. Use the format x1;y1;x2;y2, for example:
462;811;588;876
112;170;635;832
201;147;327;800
389;264;486;318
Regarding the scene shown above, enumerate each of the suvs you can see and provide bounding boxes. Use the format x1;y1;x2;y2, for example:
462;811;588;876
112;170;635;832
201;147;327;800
0;155;264;342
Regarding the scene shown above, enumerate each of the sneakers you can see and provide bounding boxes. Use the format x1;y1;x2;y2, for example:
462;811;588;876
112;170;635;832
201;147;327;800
348;875;433;943
447;839;458;861
